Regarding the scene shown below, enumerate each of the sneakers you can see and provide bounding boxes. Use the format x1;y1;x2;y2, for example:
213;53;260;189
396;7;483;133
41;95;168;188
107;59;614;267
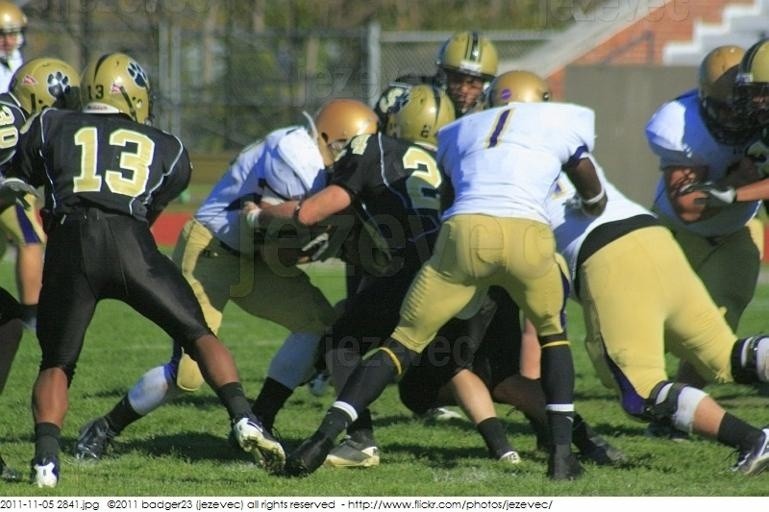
284;438;328;477
29;454;59;489
494;447;520;466
721;428;769;476
73;417;105;460
0;455;24;480
324;441;380;469
235;412;287;473
576;446;623;467
548;455;586;482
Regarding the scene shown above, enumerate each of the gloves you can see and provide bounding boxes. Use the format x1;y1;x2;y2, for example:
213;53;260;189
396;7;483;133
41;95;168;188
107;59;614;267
678;181;736;209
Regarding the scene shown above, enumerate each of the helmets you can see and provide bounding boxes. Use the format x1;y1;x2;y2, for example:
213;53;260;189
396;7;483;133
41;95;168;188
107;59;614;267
307;98;383;168
698;45;760;144
384;86;455;146
433;31;499;80
734;40;769;140
482;70;555;110
0;1;28;31
8;56;82;114
79;52;163;127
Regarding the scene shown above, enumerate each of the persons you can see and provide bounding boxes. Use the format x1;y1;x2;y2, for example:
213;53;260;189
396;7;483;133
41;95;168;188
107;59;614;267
547;154;769;480
598;46;766;438
0;57;83;399
270;69;608;481
0;50;287;485
681;40;768;202
244;83;522;470
405;31;621;467
71;99;384;462
1;1;49;330
